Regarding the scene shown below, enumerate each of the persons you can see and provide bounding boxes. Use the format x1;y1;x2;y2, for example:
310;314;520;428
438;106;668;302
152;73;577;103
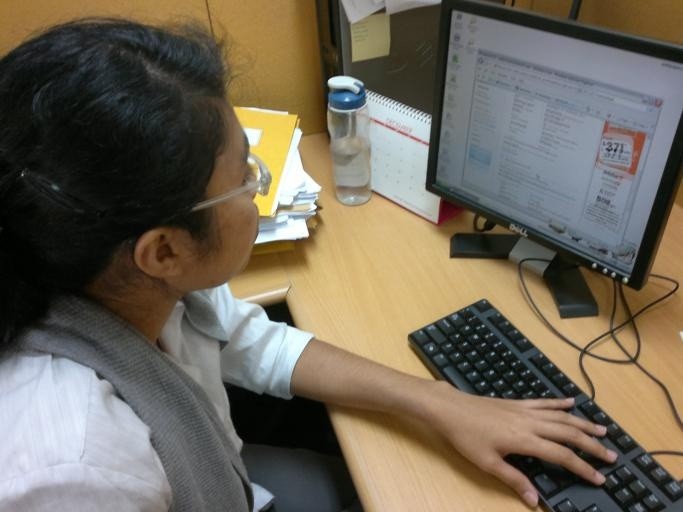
0;16;618;512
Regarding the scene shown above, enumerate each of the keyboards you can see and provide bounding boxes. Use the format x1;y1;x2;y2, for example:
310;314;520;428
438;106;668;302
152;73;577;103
407;298;682;512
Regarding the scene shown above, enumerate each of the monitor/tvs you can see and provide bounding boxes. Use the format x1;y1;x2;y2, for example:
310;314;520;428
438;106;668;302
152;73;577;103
425;1;683;320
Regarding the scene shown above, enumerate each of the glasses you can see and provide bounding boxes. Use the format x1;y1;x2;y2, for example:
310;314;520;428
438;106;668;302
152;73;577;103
189;151;272;214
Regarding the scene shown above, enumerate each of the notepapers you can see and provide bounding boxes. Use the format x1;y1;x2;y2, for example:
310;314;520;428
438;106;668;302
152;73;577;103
350;13;391;63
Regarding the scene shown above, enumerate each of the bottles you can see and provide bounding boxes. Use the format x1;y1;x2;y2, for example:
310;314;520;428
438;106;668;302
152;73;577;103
326;77;372;206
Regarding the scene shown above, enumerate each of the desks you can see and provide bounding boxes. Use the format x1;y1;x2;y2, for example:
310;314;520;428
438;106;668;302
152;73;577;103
226;130;682;511
